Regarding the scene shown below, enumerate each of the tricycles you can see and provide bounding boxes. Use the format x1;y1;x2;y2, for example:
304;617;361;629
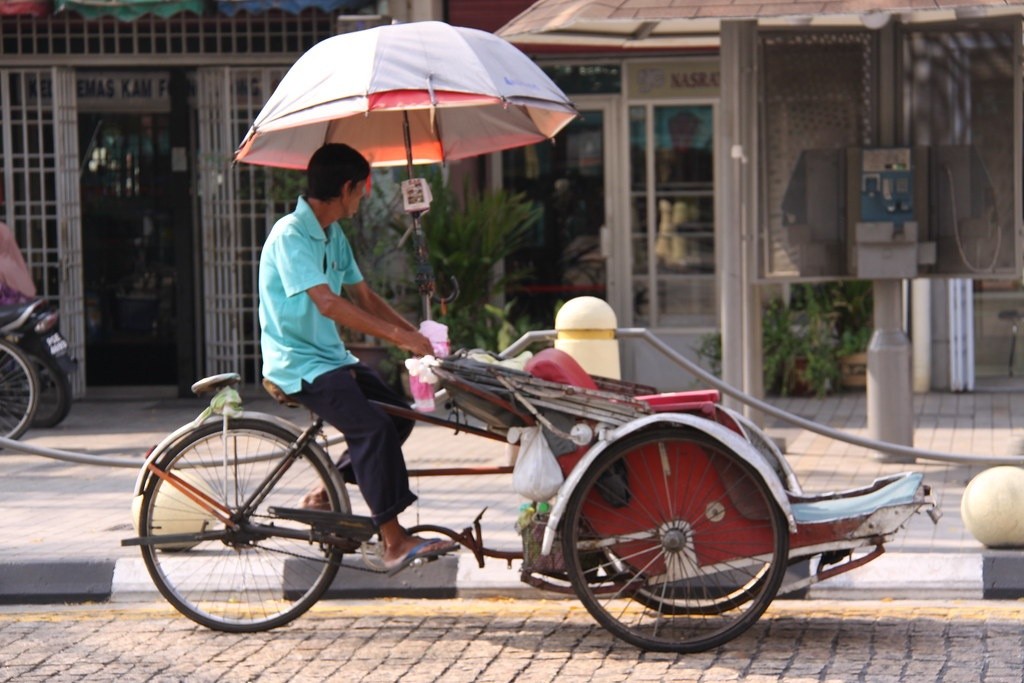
0;226;73;446
124;296;935;658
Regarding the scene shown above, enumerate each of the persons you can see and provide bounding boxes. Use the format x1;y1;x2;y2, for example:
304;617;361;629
257;140;462;579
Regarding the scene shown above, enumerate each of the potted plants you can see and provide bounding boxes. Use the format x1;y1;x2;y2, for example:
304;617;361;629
694;284;841;400
814;279;872;388
272;168;402;381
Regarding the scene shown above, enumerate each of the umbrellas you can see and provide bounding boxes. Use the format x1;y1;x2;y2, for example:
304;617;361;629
227;21;573;152
231;88;581;167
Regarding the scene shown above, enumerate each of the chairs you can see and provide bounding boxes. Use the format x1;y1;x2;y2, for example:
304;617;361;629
521;347;722;417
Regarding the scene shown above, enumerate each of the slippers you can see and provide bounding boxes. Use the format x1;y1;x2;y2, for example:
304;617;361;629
387;538;461;577
